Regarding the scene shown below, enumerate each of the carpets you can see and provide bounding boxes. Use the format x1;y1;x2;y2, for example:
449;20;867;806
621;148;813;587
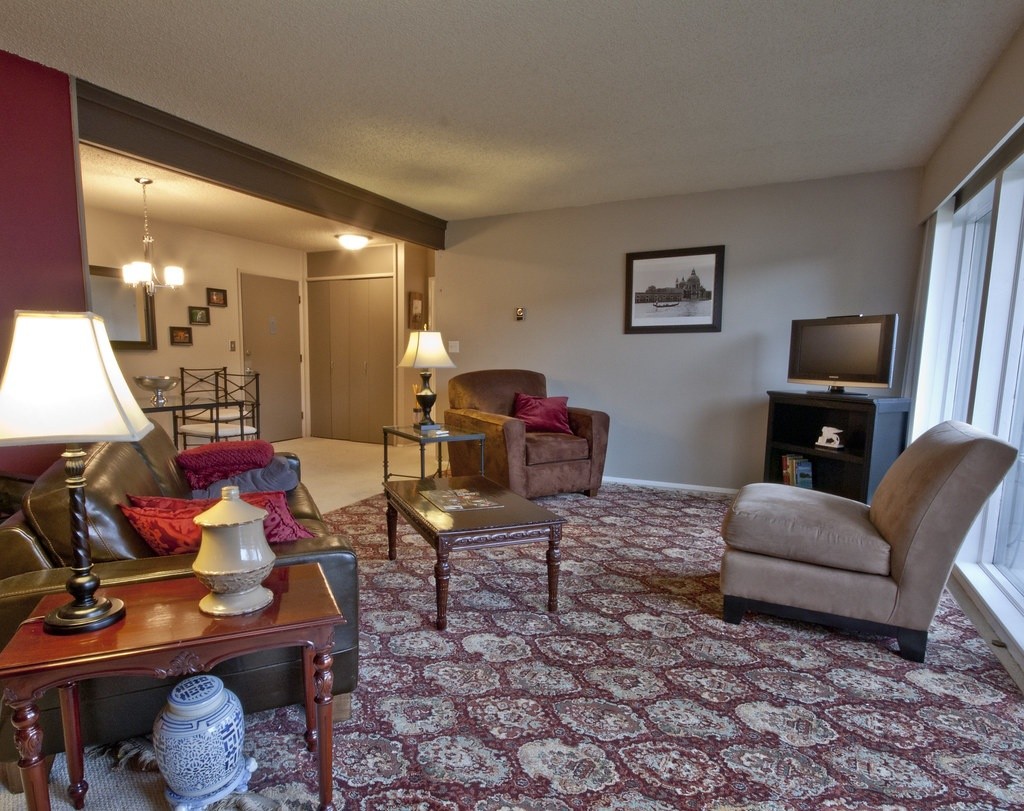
85;481;1024;811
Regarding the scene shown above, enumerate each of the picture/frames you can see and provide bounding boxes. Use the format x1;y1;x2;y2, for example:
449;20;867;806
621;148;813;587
624;244;725;334
170;327;193;346
206;287;228;308
188;306;211;325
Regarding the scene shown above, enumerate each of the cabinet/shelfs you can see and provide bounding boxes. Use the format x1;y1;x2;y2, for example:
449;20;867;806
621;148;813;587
764;389;912;506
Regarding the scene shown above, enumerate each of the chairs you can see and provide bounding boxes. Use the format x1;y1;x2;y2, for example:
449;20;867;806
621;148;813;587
177;367;261;451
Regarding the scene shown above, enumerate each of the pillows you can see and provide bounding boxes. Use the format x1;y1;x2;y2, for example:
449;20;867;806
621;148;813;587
117;456;316;556
511;392;575;436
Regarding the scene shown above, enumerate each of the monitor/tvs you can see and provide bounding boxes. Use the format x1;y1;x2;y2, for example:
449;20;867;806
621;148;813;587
787;313;898;398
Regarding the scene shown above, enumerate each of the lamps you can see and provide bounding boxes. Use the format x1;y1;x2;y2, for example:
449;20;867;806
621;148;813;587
0;310;155;636
123;178;184;296
335;234;373;250
396;323;457;430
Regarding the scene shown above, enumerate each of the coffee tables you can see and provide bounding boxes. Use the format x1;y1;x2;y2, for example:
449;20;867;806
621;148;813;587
0;562;347;811
382;423;487;482
382;473;568;629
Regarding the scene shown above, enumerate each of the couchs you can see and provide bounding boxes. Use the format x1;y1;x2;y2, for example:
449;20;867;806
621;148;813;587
0;416;359;797
444;368;610;500
720;419;1019;664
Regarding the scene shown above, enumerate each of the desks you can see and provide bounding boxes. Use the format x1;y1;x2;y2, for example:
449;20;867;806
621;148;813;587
131;393;245;452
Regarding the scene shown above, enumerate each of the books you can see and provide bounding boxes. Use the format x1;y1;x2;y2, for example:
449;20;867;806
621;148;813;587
782;454;812;488
419;488;504;511
